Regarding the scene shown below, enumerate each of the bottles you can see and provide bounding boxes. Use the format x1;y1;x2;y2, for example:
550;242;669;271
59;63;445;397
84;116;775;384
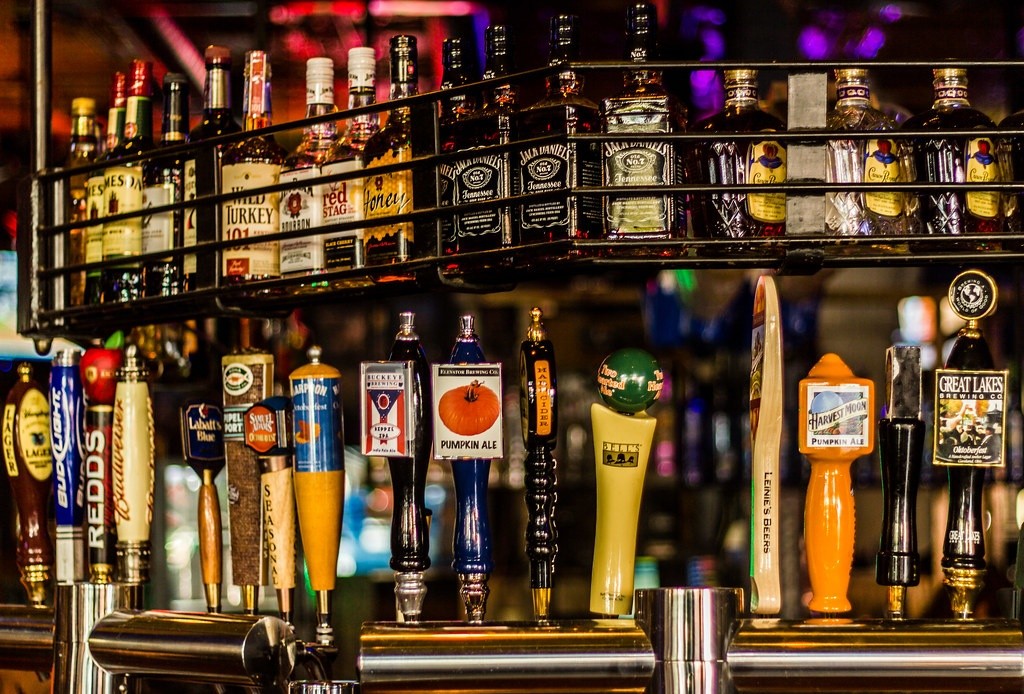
55;2;1024;307
